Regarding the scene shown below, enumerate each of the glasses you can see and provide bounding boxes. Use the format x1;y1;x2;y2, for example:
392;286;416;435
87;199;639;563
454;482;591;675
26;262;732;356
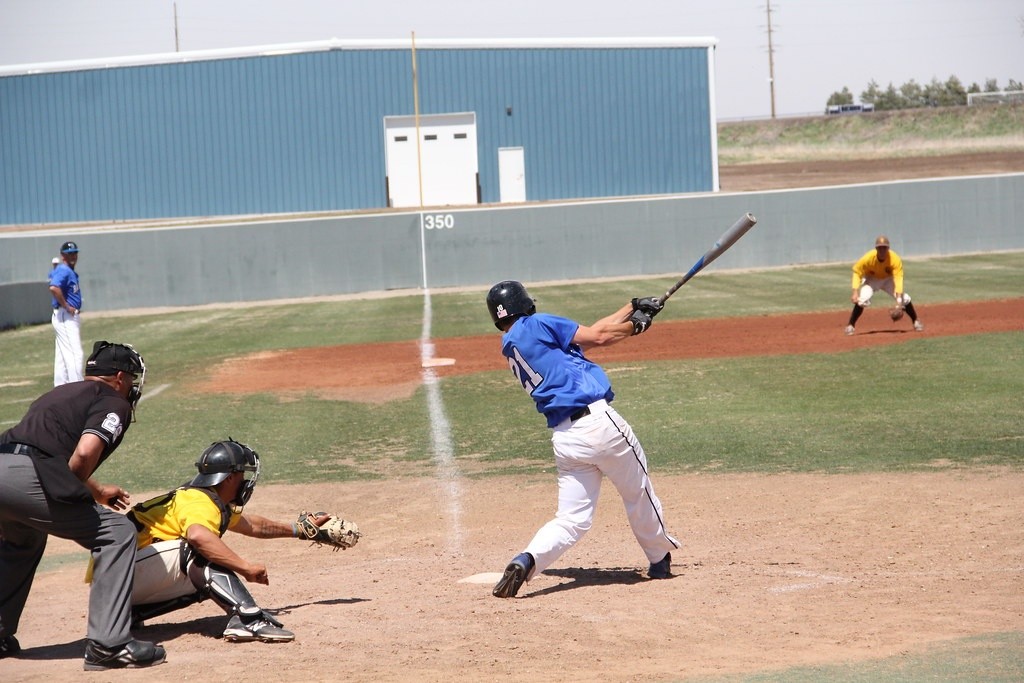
122;371;138;379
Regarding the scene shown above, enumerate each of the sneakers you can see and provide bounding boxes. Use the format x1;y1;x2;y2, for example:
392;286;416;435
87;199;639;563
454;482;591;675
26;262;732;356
223;615;295;642
0;636;20;658
83;639;166;670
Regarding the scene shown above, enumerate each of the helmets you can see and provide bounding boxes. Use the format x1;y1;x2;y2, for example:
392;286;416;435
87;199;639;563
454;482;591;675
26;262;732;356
487;281;536;331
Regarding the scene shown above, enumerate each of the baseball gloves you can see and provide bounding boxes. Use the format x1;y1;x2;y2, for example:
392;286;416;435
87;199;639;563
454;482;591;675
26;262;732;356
891;307;903;320
298;509;361;550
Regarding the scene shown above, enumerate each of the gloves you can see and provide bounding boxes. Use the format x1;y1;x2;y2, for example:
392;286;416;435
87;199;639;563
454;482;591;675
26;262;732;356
632;296;664;312
629;310;651;336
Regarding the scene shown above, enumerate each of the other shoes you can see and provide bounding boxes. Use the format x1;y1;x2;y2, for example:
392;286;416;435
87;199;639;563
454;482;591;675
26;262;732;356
913;321;923;331
493;553;530;598
845;326;855;335
647;552;671;578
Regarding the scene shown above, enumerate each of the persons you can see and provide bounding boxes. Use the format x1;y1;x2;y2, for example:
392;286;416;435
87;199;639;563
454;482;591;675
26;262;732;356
86;436;362;643
485;280;680;598
48;242;86;389
0;340;168;672
845;236;924;336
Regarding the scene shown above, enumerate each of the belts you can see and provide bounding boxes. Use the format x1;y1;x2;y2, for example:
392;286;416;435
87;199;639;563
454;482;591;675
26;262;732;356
0;443;27;455
570;406;591;421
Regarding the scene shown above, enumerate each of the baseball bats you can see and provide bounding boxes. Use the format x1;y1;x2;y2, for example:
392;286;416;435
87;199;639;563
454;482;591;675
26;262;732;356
51;257;61;270
657;211;758;305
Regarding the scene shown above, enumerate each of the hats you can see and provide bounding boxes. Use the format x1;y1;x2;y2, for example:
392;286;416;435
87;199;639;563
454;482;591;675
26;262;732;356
52;258;59;263
190;441;254;486
85;341;143;376
61;242;80;252
875;237;889;247
